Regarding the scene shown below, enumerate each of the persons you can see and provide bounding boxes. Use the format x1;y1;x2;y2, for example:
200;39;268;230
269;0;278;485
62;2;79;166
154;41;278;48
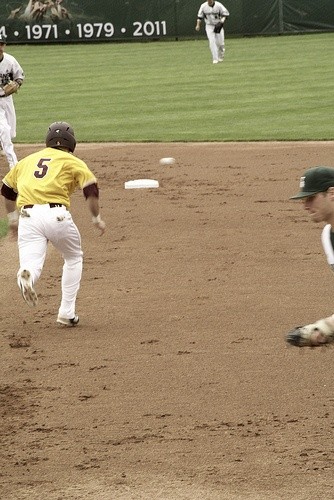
0;34;24;171
1;121;106;327
288;166;334;348
196;0;229;63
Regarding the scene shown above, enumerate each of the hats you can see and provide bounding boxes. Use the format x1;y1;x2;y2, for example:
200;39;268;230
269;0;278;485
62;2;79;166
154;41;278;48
0;33;7;44
288;165;334;200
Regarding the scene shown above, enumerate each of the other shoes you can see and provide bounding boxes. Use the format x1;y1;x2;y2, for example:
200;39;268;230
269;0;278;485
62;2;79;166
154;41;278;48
213;57;223;64
18;269;38;307
57;314;79;327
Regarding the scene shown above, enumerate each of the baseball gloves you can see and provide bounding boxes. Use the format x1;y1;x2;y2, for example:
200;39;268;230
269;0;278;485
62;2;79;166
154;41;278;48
0;78;23;98
213;22;225;34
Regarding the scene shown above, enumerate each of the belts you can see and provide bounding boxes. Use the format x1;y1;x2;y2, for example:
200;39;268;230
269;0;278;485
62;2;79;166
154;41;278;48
23;203;62;209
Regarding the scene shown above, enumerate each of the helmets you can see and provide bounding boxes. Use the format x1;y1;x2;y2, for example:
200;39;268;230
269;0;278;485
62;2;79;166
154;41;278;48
45;120;77;153
